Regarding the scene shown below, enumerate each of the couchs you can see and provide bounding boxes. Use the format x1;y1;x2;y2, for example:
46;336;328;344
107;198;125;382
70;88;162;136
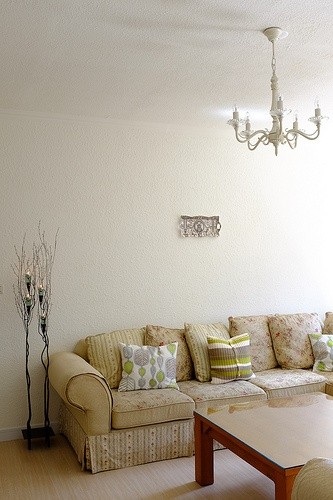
44;311;333;475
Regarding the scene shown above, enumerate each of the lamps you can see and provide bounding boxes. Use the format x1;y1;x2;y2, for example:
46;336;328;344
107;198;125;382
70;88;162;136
225;28;328;154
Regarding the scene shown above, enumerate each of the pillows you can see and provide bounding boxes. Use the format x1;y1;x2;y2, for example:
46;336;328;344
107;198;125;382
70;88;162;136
115;342;179;391
228;312;279;372
309;334;333;372
86;326;146;389
181;322;231;383
143;324;194;384
205;333;256;385
268;313;326;371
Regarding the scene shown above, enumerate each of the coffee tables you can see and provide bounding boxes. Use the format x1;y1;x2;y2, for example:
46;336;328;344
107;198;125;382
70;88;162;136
193;391;333;500
